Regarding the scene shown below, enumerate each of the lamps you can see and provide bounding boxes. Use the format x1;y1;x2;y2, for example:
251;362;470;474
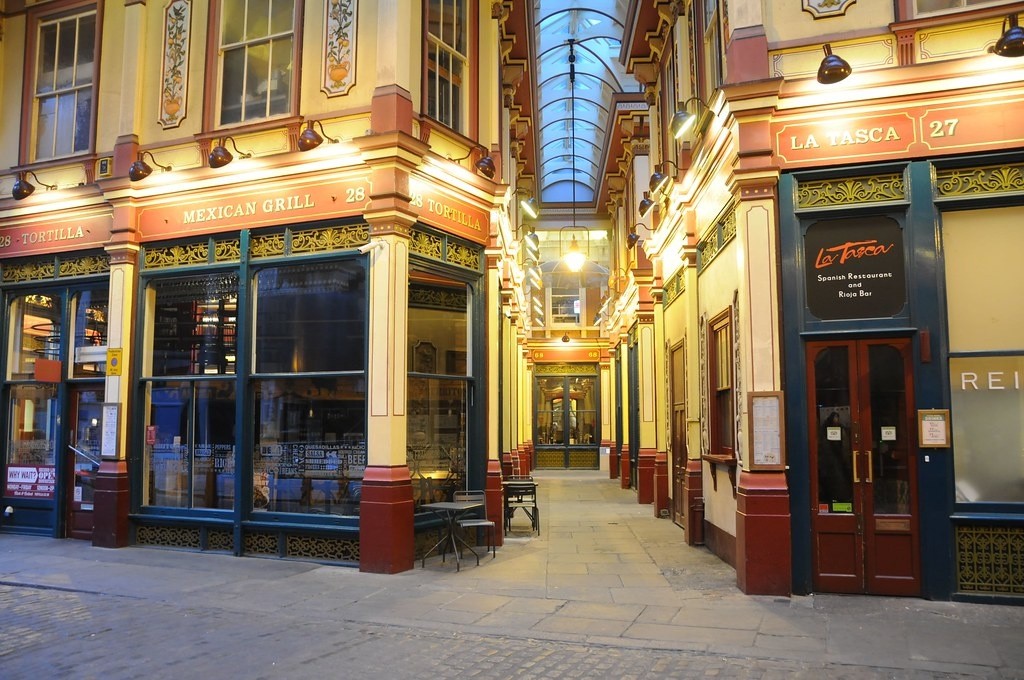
298;119;340;153
817;44;853;84
608;267;626;288
12;170;58;202
561;332;570;343
595;301;616;313
592;310;611;326
600;288;619;306
626;223;657;250
649;160;689;193
512;186;540;219
987;11;1024;57
448;145;497;180
510;223;546;326
128;151;172;181
670;96;710;139
208;136;253;168
559;38;590;272
638;188;667;219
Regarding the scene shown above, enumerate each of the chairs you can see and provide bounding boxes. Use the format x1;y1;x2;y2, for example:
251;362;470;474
427;477;465;503
413;477;426;514
505;474;541;536
453;490;496;560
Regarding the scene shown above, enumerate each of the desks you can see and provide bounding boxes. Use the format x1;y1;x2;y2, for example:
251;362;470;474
196;467;363;514
501;481;539;530
418;502;484;572
